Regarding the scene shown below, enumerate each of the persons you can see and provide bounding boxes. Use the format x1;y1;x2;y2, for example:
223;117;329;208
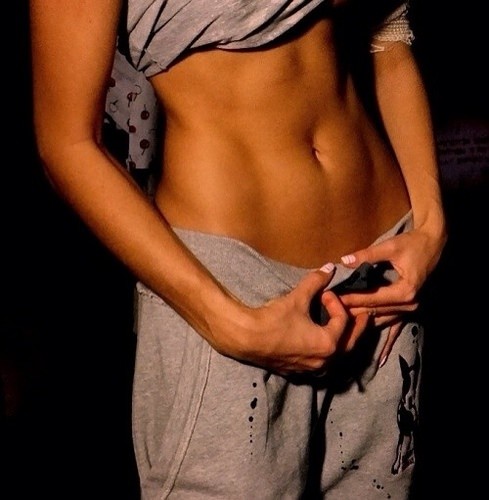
29;0;449;500
